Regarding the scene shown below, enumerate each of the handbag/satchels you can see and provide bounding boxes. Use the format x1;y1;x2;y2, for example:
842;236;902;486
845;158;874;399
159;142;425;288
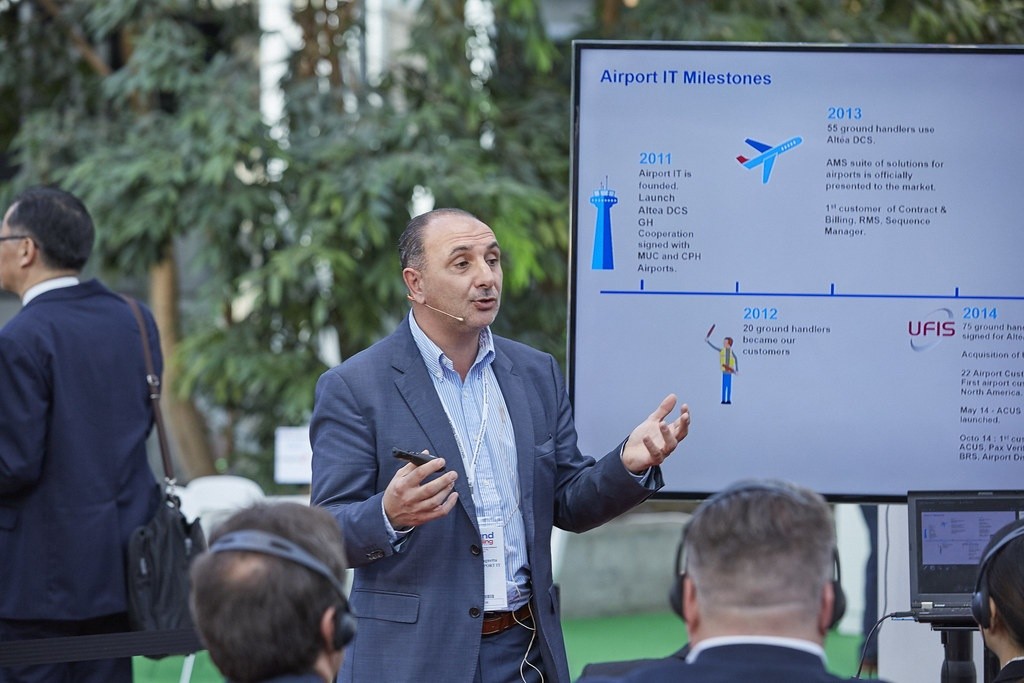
126;491;210;659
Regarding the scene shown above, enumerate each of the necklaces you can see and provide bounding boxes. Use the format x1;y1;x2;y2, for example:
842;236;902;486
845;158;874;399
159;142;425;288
20;274;77;303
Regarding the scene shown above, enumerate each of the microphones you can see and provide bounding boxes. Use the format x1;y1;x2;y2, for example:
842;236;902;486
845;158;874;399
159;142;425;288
424;304;463;322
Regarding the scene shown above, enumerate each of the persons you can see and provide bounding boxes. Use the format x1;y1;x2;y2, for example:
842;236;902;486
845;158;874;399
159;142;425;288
191;502;347;683
576;480;890;683
309;208;691;683
0;190;163;683
979;519;1024;683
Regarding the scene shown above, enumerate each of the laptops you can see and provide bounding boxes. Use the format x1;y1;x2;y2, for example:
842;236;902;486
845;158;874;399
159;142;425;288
907;489;1024;621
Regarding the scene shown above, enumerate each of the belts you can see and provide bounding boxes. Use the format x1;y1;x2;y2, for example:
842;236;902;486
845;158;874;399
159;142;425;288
478;606;534;637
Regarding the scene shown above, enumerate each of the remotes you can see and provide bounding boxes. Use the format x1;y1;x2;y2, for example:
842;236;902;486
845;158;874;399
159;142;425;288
392;446;447;473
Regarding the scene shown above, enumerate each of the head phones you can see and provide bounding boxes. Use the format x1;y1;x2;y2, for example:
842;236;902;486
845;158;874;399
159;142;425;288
210;529;357;650
972;527;1024;628
669;482;847;629
